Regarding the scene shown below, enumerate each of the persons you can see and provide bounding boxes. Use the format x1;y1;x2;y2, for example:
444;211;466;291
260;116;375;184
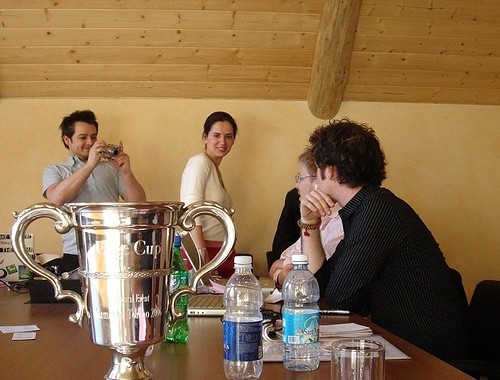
42;110;146;303
265;148;345;292
179;111;237;279
297;118;476;363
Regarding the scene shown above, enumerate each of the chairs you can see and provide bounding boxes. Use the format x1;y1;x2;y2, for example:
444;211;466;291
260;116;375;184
469;280;500;380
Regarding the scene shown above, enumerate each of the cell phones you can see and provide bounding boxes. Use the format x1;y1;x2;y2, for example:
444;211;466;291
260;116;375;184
319;309;350;314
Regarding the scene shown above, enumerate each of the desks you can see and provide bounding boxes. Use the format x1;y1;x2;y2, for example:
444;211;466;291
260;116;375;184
0;276;477;380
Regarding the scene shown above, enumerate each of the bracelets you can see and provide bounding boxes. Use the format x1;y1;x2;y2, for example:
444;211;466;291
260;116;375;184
297;218;322;237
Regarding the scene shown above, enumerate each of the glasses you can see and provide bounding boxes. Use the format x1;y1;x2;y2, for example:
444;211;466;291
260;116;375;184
295;174;317;183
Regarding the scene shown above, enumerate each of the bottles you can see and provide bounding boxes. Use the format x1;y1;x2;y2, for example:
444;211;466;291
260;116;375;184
282;254;320;371
331;339;385;380
224;256;264;380
167;236;190;342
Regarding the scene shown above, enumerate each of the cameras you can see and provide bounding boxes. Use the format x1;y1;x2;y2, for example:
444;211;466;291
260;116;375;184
102;144;119;158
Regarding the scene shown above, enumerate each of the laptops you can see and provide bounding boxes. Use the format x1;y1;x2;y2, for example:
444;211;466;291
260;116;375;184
176;230;275;293
185;294;227;315
